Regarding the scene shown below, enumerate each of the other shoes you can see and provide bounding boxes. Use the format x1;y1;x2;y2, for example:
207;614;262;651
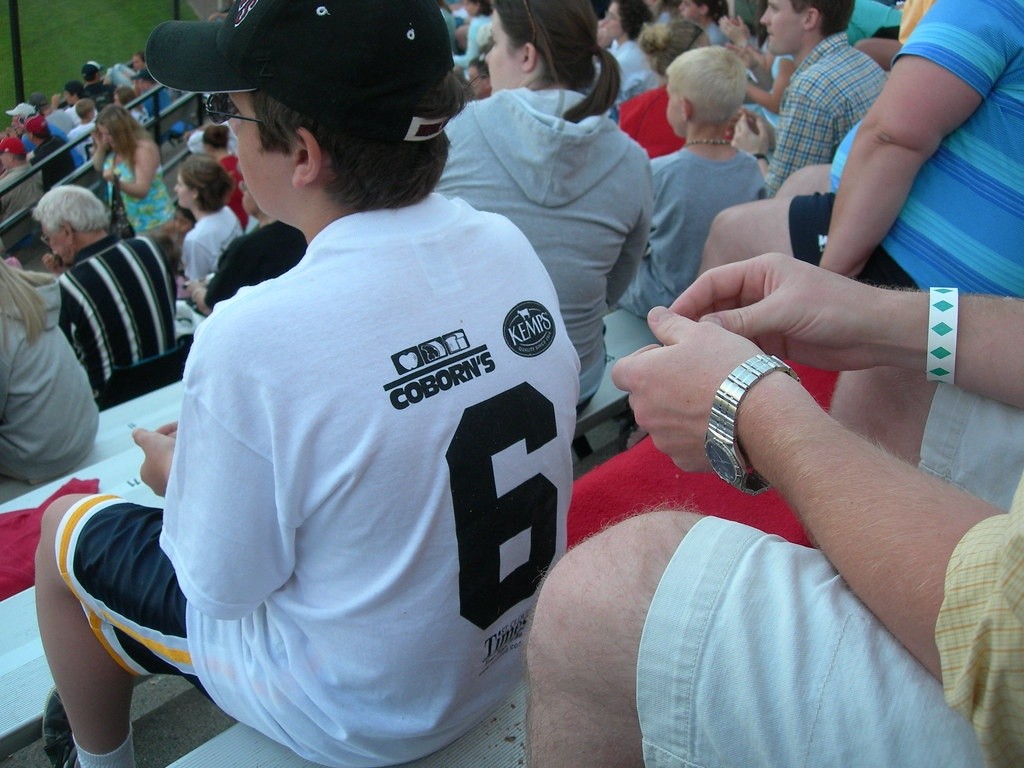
39;687;80;768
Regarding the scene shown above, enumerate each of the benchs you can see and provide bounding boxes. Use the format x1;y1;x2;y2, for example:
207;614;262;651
0;303;672;768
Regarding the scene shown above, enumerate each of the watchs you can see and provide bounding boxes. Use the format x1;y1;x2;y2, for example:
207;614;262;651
704;355;801;495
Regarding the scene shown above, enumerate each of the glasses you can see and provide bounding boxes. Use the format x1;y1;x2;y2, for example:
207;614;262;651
205;92;262;125
40;226;62;246
603;11;622;24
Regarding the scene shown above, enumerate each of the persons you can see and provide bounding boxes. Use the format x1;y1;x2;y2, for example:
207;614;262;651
36;0;580;768
611;46;764;319
526;252;1024;768
697;0;1024;298
0;52;308;483
435;0;656;416
446;1;494;100
596;0;935;198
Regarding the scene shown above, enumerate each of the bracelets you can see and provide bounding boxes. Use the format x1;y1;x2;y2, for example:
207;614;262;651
926;287;959;385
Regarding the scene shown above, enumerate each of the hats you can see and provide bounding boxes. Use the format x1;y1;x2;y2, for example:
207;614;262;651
24;114;49;137
81;61;104;78
0;135;26;154
147;0;461;142
130;67;154;81
4;103;36;119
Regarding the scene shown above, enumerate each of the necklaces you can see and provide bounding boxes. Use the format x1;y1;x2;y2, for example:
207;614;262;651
685;139;730;145
